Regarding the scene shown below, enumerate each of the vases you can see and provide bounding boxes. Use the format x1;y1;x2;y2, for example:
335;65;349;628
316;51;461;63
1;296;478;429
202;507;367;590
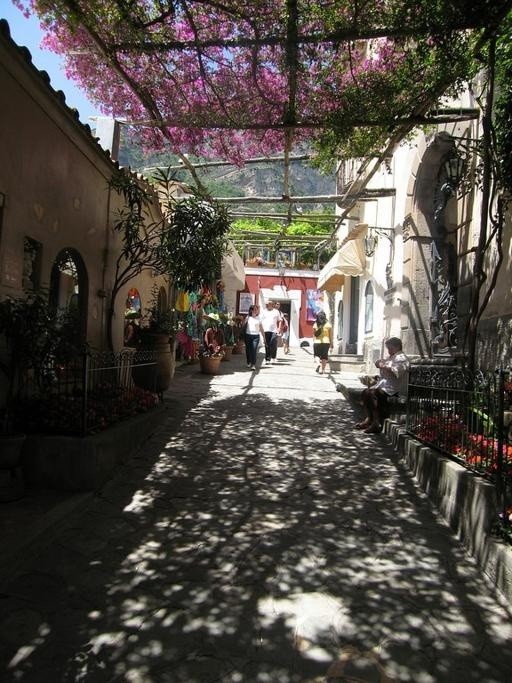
222;346;234;361
234;343;245;353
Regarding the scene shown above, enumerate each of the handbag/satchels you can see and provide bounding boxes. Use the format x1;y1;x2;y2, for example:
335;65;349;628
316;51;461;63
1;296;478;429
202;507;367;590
241;322;249;336
313;328;322;337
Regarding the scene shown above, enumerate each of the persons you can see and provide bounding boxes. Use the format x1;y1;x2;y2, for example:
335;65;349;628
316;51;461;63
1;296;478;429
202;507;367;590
240;299;290;371
311;310;335;374
353;336;411;433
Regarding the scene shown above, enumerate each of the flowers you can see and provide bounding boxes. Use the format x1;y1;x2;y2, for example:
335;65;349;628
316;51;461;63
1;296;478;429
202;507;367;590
220;313;230;346
232;316;242;342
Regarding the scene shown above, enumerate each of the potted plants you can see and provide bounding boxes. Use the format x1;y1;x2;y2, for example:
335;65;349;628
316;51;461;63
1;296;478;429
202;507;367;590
133;303;177;390
198;344;222;373
